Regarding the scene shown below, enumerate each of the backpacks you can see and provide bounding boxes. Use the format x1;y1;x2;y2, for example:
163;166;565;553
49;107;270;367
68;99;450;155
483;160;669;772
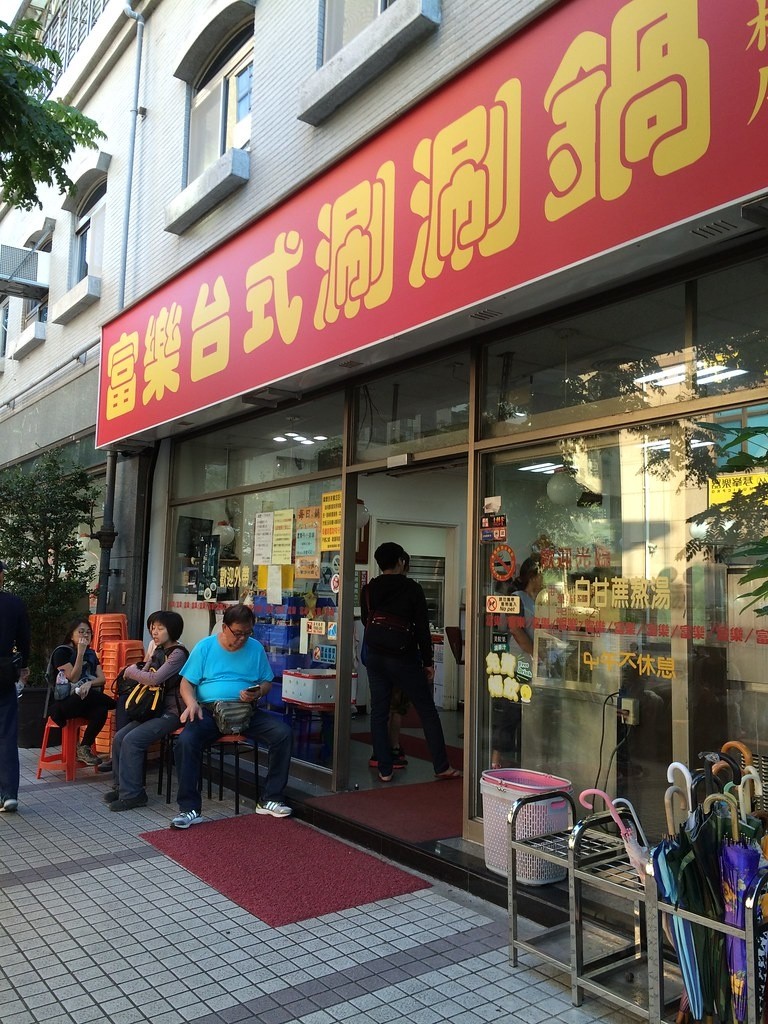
125;654;167;724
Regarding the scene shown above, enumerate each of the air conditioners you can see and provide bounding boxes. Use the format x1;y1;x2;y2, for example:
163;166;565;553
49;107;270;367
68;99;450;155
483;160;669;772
0;242;51;301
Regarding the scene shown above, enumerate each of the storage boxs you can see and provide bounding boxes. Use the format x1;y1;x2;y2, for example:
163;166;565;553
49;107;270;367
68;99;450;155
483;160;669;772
267;654;312;678
282;668;358;710
260;682;286;707
254;623;313;649
252;593;336;621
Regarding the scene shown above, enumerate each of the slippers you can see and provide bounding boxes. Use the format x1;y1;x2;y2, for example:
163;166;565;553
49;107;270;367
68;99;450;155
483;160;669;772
378;772;393;782
434;769;463;777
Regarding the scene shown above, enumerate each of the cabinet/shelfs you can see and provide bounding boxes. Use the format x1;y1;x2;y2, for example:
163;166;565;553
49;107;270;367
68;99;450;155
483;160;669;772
506;791;768;1024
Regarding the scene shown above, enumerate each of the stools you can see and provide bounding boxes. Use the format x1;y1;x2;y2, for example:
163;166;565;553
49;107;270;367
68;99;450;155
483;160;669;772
37;612;184;806
203;734;260;815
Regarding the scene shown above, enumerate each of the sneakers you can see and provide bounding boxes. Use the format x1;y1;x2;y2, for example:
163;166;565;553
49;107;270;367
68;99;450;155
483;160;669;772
109;788;148;811
76;745;102;765
170;809;204;828
369;756;408;767
103;790;119;802
393;751;406;759
255;800;292;817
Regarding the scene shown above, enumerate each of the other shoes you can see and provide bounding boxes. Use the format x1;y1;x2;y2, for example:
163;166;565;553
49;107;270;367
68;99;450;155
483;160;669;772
0;799;17;812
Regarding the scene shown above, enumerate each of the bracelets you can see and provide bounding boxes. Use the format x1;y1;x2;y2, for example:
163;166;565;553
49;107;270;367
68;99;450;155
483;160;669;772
254;689;261;700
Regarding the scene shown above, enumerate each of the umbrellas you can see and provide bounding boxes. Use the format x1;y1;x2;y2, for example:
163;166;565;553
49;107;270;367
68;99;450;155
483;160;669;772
579;741;768;1024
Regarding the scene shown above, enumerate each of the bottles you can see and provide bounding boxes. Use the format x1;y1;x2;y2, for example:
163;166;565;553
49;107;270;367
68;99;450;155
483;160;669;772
55;668;66;699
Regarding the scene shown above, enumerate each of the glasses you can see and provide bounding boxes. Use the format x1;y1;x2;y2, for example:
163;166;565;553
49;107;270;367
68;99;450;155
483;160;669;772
74;629;94;635
227;624;254;639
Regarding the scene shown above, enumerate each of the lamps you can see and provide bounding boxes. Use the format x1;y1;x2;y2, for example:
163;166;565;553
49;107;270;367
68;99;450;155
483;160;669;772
546;455;589;509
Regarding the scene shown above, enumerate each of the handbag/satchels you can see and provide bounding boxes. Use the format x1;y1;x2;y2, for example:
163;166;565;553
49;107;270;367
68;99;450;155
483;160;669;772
203;700;257;734
361;584;416;658
111;662;146;696
44;646;102;700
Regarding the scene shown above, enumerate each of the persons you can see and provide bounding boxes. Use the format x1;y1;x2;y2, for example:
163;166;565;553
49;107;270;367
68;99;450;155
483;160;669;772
98;611;161;772
556;629;742;779
368;550;410;769
492;554;546;768
0;563;30;812
170;604;294;828
361;542;462;783
103;610;190;812
45;618;116;766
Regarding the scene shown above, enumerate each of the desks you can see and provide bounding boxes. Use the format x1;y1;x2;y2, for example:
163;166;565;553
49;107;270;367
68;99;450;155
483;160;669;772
282;701;335;767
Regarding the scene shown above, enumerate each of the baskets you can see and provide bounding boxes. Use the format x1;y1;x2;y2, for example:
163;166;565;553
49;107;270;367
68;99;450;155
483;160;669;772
480;768;574;886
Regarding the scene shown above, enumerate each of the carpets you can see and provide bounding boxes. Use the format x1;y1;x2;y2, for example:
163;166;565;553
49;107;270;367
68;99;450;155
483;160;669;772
300;778;463;844
138;814;434;930
346;730;464;771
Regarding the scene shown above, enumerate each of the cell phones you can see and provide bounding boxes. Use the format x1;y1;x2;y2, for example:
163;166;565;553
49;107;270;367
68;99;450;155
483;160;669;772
246;685;260;692
